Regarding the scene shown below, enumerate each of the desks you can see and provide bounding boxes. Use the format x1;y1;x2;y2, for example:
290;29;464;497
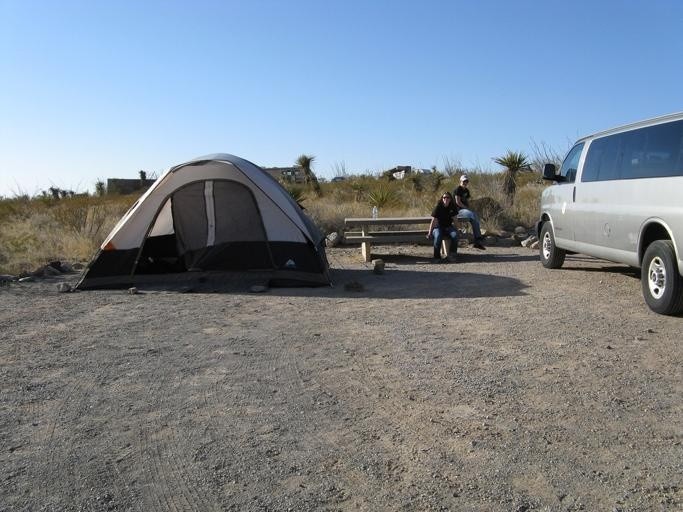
345;216;471;262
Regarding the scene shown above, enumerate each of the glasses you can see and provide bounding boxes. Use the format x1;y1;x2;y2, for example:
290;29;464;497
443;196;451;200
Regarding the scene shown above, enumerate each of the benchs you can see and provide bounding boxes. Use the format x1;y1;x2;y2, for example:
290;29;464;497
343;230;473;263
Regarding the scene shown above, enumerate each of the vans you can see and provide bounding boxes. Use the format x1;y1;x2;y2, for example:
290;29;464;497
534;110;683;316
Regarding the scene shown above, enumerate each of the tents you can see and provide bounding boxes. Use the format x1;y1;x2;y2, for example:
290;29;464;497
75;152;335;290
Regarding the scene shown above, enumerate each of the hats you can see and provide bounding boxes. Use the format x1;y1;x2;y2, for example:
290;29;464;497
459;175;469;182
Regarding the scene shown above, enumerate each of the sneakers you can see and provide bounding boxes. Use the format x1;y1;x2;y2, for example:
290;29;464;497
473;243;485;249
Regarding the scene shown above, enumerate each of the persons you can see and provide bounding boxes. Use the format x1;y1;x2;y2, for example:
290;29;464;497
426;191;459;264
453;174;487;251
